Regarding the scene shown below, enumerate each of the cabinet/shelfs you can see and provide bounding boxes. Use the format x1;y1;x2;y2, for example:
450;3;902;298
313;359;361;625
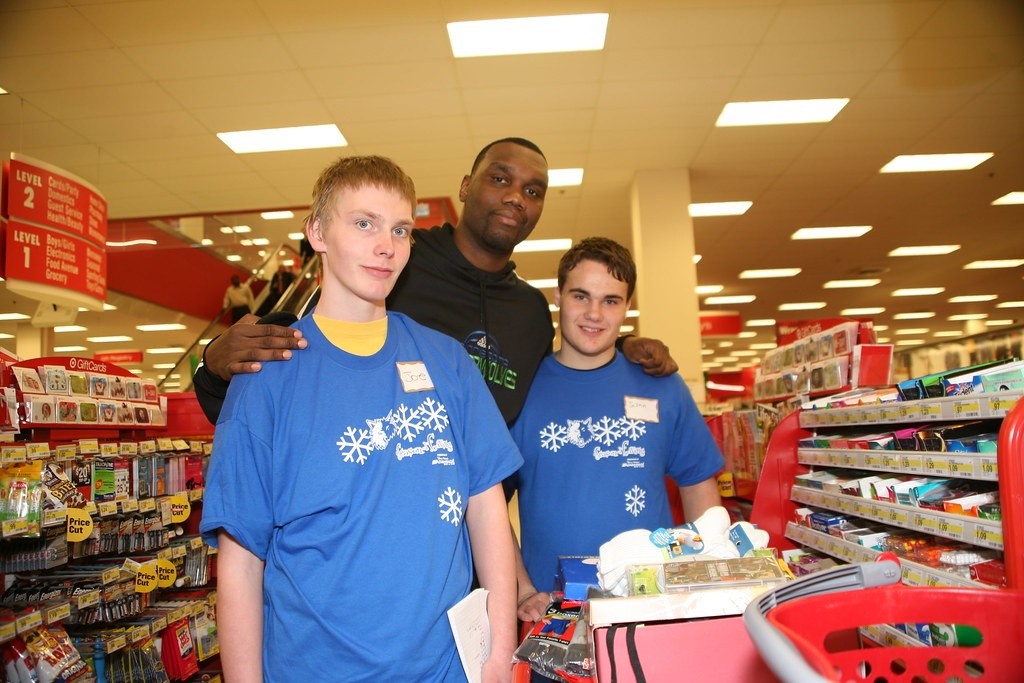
784;390;1024;683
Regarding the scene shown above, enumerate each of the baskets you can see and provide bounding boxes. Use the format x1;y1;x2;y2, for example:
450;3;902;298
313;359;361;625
741;552;1024;683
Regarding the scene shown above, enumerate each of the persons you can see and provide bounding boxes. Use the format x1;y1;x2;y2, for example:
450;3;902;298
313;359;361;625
192;136;680;593
506;233;727;648
198;153;521;683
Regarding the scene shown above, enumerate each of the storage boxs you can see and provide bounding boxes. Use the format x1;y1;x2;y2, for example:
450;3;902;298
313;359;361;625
721;409;764;482
557;555;601;601
